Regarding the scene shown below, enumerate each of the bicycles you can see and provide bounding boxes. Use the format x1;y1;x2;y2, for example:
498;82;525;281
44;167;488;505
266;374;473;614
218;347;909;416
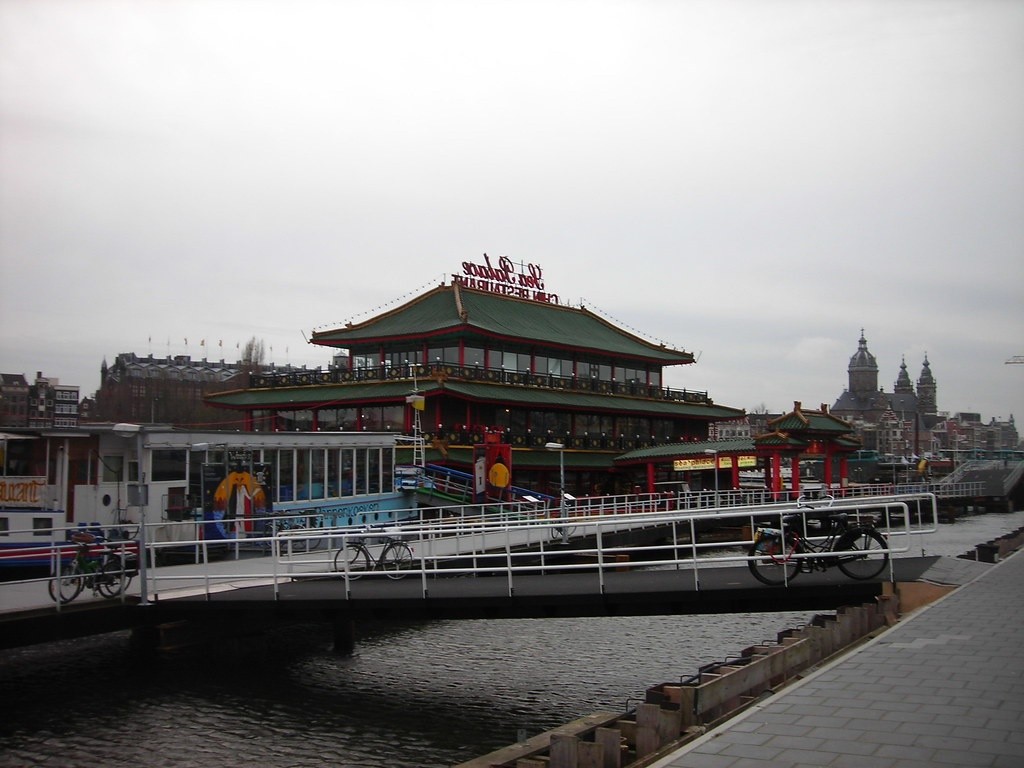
745;492;890;585
264;509;322;553
49;531;142;604
332;533;415;580
